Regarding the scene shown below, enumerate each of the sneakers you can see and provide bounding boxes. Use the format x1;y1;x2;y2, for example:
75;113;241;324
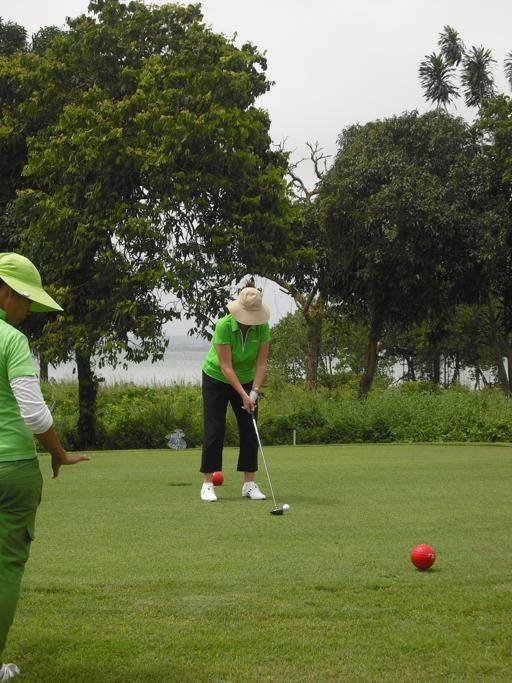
0;663;20;682
200;486;217;502
242;485;266;500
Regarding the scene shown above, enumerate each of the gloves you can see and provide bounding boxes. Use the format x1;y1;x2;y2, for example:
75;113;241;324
241;391;258;410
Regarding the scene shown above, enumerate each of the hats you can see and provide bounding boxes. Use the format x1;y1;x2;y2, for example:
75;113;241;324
225;288;269;325
0;252;64;313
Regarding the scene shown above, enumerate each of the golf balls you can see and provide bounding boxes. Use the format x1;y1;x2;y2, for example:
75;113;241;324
213;472;225;485
411;544;436;571
282;504;290;510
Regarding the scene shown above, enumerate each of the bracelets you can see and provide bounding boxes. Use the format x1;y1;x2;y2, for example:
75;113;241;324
248;390;259;405
253;387;259;391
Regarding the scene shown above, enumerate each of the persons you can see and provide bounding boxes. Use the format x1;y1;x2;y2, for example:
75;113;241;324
0;249;91;683
198;287;272;502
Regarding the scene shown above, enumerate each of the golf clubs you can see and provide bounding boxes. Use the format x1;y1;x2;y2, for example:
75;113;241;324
250;408;284;515
